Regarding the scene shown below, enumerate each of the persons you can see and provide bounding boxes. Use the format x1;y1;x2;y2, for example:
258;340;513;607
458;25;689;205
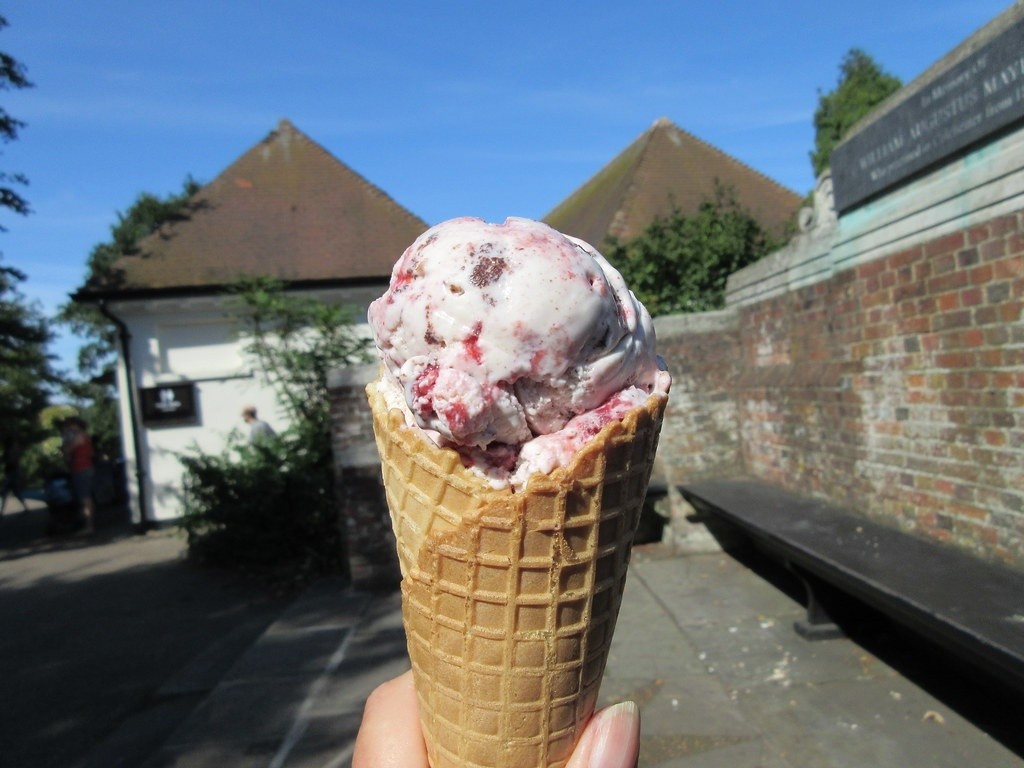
242;406;275;442
60;417;96;537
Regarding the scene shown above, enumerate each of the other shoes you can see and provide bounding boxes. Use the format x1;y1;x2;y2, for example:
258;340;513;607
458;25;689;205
73;527;96;537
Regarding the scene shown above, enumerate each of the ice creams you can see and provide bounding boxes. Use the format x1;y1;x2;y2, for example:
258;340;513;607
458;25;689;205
364;216;672;768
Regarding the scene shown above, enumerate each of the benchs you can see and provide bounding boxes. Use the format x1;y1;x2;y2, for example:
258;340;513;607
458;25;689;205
675;476;1024;686
